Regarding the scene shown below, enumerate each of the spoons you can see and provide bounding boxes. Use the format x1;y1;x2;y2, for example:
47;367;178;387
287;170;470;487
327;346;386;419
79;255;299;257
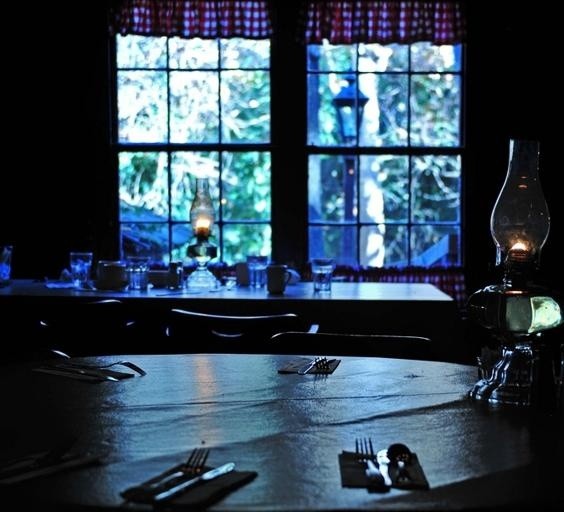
386;443;412;486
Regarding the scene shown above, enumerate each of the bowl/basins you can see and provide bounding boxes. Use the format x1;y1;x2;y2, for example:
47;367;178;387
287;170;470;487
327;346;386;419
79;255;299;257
148;271;168;288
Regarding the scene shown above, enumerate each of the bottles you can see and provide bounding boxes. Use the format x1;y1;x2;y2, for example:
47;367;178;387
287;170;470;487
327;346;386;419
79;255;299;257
167;261;184;292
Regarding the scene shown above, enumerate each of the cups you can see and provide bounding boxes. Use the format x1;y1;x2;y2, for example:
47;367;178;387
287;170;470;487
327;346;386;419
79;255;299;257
266;264;292;296
70;252;93;291
245;255;268;289
310;257;337;294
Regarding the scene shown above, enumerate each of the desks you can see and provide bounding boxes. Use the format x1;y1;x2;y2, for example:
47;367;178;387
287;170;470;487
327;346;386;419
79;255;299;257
0;277;563;511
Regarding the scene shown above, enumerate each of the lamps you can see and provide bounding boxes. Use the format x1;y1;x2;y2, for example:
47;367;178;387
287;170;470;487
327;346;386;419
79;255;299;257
464;138;563;411
180;178;222;288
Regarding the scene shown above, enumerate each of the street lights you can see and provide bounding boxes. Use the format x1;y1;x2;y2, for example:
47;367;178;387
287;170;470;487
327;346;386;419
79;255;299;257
329;65;373;268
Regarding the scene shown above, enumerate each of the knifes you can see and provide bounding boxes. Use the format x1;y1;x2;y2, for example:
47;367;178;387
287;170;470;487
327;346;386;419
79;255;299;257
31;366;120;383
153;461;235;502
297;356;326;375
376;448;392;487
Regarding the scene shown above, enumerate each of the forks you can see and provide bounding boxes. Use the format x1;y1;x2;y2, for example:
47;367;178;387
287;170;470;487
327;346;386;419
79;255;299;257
63;360;147;376
148;448;211;489
314;357;336;369
355;437;385;486
